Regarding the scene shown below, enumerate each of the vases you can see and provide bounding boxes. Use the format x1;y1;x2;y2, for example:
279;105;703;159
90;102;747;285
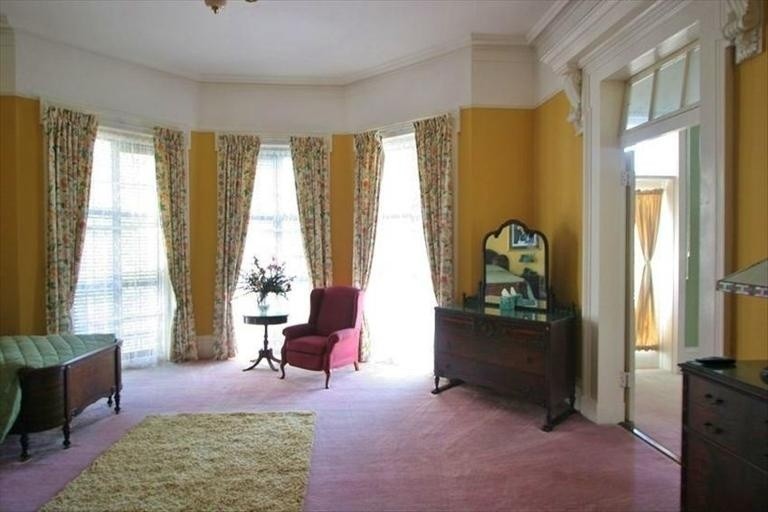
256;300;270;316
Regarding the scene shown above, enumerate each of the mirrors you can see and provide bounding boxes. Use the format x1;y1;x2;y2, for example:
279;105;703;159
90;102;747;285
480;218;551;314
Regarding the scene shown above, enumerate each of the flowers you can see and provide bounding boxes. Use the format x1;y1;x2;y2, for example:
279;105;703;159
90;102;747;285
237;253;300;301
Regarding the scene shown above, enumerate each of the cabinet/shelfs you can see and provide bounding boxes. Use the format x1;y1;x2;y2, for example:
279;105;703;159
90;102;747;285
431;296;579;433
675;354;768;511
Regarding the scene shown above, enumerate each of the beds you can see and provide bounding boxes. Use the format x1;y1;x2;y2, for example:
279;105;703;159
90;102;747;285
1;334;125;462
483;248;536;301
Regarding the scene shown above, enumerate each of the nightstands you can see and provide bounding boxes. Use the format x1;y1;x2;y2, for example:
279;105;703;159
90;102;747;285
520;274;545;299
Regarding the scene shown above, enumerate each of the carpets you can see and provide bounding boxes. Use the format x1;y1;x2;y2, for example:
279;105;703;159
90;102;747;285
34;410;315;512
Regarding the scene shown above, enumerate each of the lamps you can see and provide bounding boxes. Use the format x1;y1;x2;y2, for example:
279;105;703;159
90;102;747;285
205;0;257;17
518;254;534;274
713;258;768;378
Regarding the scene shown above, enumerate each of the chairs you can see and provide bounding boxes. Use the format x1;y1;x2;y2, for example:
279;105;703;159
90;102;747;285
279;286;365;391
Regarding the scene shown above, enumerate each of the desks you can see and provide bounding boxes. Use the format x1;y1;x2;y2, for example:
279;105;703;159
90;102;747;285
242;313;288;374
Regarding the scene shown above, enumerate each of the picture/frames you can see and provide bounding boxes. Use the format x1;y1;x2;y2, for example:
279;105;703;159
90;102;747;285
508;223;540;250
